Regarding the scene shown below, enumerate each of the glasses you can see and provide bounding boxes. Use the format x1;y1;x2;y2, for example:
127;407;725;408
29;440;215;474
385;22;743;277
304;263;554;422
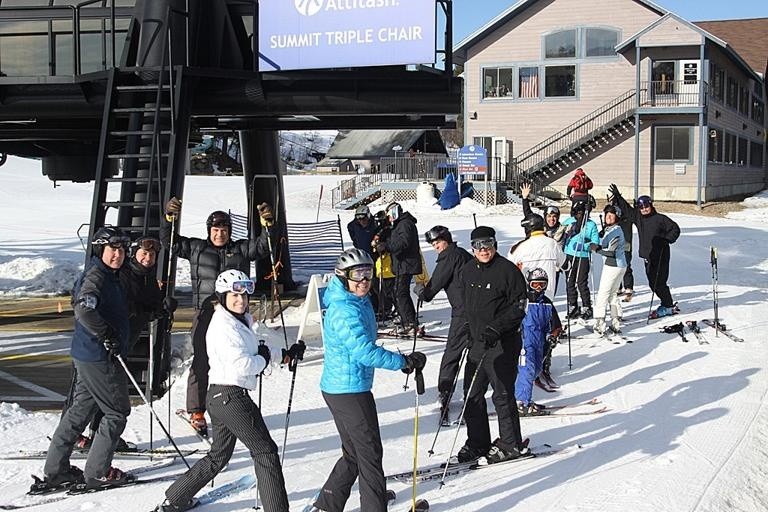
426;229;446;242
335;267;374;282
546;206;559;215
528;281;547;290
215;280;255;294
470;236;495;251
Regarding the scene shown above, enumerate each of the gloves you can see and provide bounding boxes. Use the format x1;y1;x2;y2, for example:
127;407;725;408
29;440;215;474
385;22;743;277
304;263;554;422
258;345;271;366
477;327;501;350
164;197;182;222
162;296;179;312
257;202;274;227
413;283;426;297
608;183;622;199
376;242;392;254
102;337;121;357
403;350;426;374
281;340;306;368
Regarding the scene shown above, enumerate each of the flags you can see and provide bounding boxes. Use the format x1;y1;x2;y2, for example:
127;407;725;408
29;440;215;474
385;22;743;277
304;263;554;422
519;67;538;98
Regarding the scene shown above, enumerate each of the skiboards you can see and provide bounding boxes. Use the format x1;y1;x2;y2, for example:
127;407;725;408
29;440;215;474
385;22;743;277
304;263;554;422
687;319;744;346
587;319;633;345
487;399;606;419
302;490;429;512
637;304;681;325
375;320;442;339
535;373;559;392
1;458;228;511
0;448;199;461
148;474;257;512
616;292;635;302
385;444;582;484
561;308;592;331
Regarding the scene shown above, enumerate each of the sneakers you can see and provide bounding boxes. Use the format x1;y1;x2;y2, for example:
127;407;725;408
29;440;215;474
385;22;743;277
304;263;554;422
44;465;85;485
73;435;91;448
565;307;622;335
515;401;544;417
651;306;675;318
625;288;633;295
457;446;490;463
486;439;524;464
160;497;200;511
85;467;134;490
375;311;420;337
189;412;207;433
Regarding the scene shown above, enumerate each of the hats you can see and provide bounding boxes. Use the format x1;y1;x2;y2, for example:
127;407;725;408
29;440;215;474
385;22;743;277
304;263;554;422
470;227;498;252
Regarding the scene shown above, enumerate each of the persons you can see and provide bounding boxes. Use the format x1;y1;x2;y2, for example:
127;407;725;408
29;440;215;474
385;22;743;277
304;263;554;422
159;269;291;512
347;200;424;339
309;247;428;512
415;225;534;467
57;235;179;453
509;168;682;417
158;196;276;438
40;228;135;488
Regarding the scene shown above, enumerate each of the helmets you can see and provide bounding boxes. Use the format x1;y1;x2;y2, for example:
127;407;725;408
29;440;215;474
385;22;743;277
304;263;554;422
521;213;545;235
425;226;452;245
206;211;232;237
526;268;548;293
635;195;654;213
91;227;162;271
354;202;403;230
544;206;560;221
571;199;587;219
604;204;622;225
215;269;256;306
334;249;375;290
